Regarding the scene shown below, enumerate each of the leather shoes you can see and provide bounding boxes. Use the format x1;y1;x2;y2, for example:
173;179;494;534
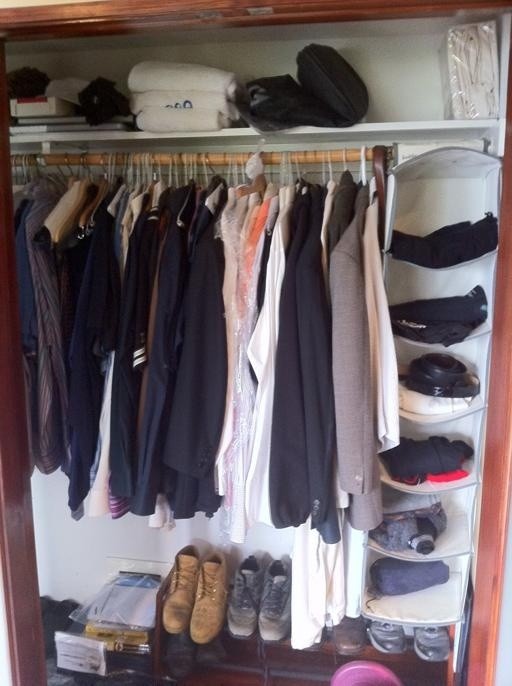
332;615;365;656
194;638;228;666
304;626;327;652
162;631;195;680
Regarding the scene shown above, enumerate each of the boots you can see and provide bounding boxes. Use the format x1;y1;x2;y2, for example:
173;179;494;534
189;550;229;645
162;544;203;635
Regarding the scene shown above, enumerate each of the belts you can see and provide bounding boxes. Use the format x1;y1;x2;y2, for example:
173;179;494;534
405;353;474;398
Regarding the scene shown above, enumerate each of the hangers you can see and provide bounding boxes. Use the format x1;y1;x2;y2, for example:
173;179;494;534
12;146;397;248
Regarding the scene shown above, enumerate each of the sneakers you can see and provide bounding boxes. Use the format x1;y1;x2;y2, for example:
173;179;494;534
226;555;265;641
258;560;291;644
413;626;451;663
366;620;408;655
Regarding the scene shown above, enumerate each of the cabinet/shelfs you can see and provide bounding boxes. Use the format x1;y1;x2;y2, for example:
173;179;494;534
1;0;510;686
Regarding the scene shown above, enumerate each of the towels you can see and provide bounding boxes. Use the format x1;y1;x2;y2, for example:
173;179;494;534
122;61;238;135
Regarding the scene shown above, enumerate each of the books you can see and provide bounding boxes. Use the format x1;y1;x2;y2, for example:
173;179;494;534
9;114;134;132
54;617;109;677
